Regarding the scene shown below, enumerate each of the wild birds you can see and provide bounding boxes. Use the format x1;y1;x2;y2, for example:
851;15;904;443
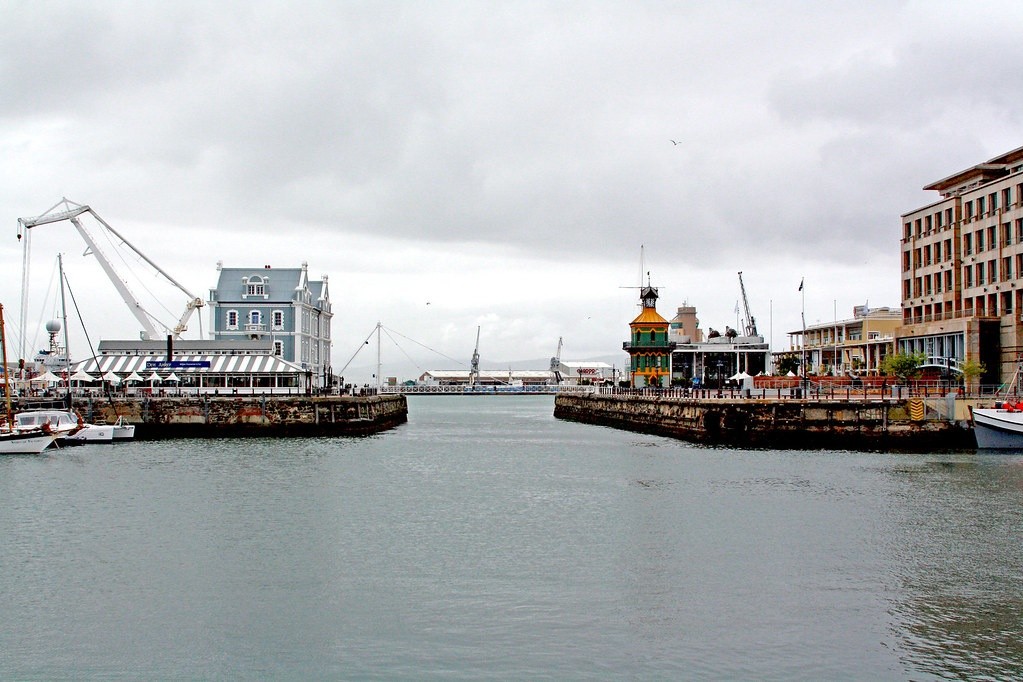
670;140;682;145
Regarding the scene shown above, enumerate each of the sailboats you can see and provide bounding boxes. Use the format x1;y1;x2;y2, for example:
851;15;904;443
0;249;137;456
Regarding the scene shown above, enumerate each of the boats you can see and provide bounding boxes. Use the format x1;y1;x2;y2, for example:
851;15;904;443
969;369;1022;452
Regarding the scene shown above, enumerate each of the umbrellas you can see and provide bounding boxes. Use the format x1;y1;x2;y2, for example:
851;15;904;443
736;371;752;380
30;371;64;397
95;370;122;398
64;368;95;397
164;372;181;397
785;370;796;376
728;374;736;380
122;370;144;397
144;372;163;397
756;371;765;377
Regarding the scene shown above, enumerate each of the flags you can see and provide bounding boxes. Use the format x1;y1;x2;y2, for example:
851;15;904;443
799;278;803;291
862;300;868;316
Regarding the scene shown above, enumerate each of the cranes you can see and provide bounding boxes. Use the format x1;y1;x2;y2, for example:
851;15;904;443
15;198;206;338
